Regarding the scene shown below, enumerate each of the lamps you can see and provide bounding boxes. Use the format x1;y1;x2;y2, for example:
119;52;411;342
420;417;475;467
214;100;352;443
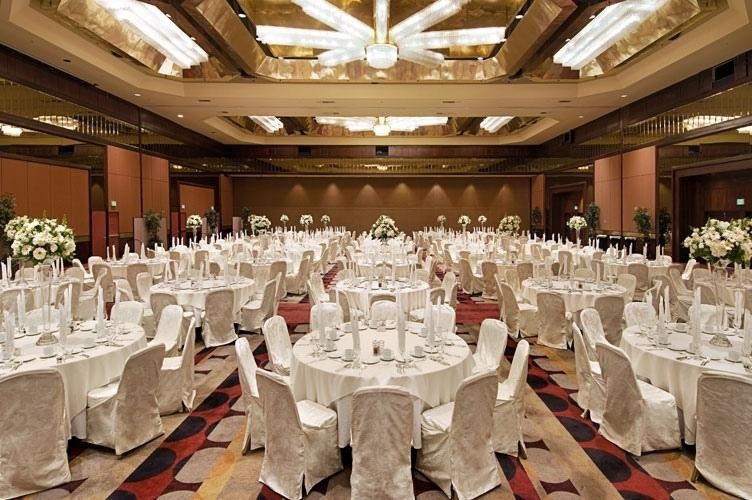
480;116;515;134
95;0;209;69
256;0;506;69
313;116;448;136
248;116;283;134
553;0;668;71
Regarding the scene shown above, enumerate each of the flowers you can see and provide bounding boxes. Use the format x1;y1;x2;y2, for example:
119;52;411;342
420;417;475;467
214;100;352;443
682;217;752;270
3;210;78;264
184;214;587;240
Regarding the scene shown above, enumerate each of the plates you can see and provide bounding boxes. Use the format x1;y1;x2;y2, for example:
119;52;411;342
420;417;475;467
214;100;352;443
536;277;610;292
0;322;117;364
353;278;412;290
323;321;441;365
647;323;747;363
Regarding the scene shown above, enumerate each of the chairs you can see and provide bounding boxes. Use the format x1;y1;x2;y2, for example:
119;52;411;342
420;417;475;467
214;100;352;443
0;231;752;500
